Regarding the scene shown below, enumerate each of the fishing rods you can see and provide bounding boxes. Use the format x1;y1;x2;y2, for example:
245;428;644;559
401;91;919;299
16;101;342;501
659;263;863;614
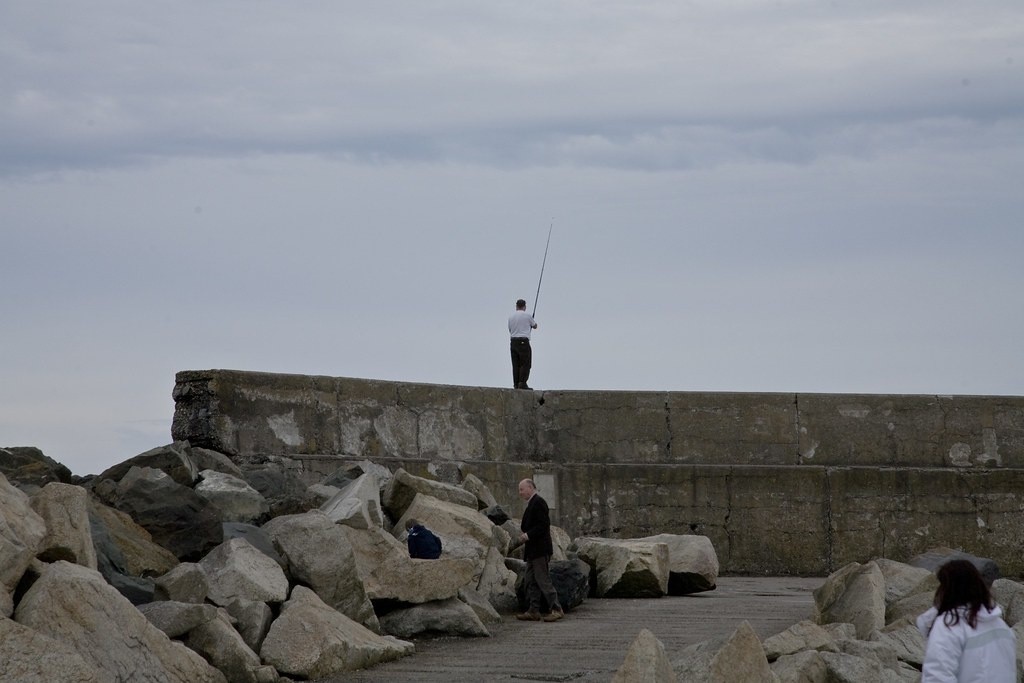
531;221;556;321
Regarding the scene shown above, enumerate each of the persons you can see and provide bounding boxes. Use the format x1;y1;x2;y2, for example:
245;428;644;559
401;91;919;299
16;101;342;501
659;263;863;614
509;300;537;390
405;518;442;559
516;478;565;621
920;560;1018;683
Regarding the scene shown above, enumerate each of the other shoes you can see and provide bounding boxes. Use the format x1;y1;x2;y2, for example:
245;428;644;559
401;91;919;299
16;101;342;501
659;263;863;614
514;382;533;390
516;610;542;621
543;610;565;621
